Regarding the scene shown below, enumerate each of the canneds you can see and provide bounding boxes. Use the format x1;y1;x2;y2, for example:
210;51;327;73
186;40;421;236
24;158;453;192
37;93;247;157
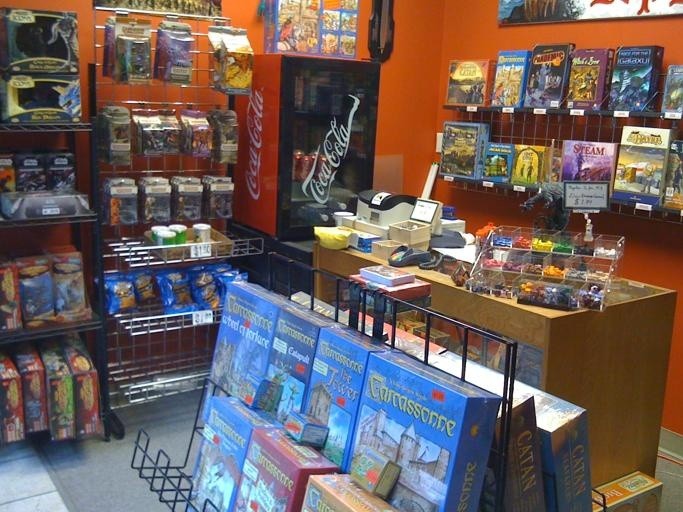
193;222;212;244
156;230;175;246
168;224;187;244
151;225;168;243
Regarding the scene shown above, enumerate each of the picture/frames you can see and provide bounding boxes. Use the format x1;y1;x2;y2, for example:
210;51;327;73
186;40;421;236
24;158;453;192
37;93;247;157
93;0;223;19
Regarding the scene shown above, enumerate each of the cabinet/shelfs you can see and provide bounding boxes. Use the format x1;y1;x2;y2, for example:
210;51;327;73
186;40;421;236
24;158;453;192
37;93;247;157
89;18;265;435
129;247;608;512
0;7;110;448
313;242;678;490
